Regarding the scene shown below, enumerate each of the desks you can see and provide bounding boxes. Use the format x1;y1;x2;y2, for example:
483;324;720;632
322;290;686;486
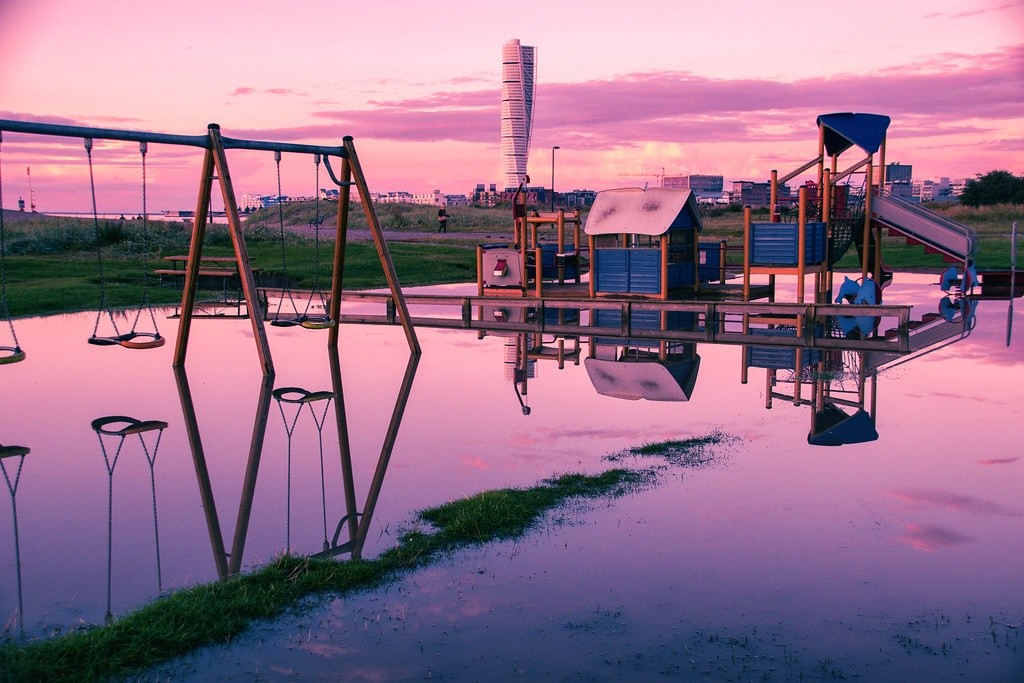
164;254;257;291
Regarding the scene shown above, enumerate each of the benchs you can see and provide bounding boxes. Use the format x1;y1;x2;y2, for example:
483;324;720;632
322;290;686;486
156;269;242;303
185;264;265;290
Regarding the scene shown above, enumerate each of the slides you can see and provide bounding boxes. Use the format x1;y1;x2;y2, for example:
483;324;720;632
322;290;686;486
851;215;893;275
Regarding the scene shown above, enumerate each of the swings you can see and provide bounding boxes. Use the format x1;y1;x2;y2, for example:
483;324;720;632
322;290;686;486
0;130;26;364
1;443;29;639
270;151;336;330
91;416;168;624
271;388;337;563
83;136;166;350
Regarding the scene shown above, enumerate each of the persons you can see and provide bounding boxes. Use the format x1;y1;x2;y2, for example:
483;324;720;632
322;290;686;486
118;214;143;220
437;204;451;234
